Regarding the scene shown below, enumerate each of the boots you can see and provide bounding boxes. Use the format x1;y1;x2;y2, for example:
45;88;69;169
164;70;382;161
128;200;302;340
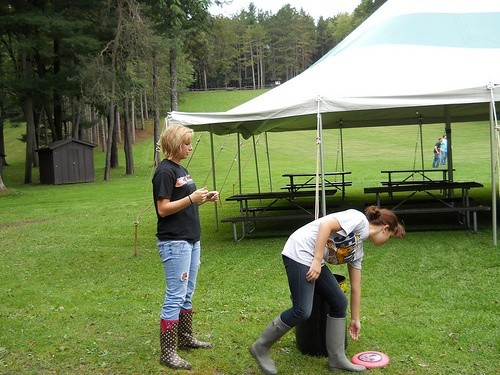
160;318;192;370
249;316;292;374
326;313;366;372
177;311;212;350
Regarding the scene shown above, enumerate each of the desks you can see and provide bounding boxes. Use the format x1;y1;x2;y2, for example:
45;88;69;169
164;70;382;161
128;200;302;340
380;168;456;194
282;173;352;195
226;189;337;236
364;181;483;231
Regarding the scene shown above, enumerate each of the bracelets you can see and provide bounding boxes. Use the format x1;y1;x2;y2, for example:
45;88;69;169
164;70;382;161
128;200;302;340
188;195;195;205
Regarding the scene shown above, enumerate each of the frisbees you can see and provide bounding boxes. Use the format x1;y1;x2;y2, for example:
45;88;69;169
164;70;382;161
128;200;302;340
351;350;391;369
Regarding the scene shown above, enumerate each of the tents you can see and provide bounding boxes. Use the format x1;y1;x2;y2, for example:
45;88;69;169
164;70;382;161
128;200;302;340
164;0;500;245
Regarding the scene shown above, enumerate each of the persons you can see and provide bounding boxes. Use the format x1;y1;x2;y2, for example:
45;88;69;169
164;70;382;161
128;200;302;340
152;124;219;369
440;136;447;165
433;138;443;167
248;206;407;375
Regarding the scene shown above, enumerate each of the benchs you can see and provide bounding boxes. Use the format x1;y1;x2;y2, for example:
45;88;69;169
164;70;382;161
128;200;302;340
282;182;352;192
380;180;449;194
221;212;330;243
238;203;316;233
365;196;491;233
